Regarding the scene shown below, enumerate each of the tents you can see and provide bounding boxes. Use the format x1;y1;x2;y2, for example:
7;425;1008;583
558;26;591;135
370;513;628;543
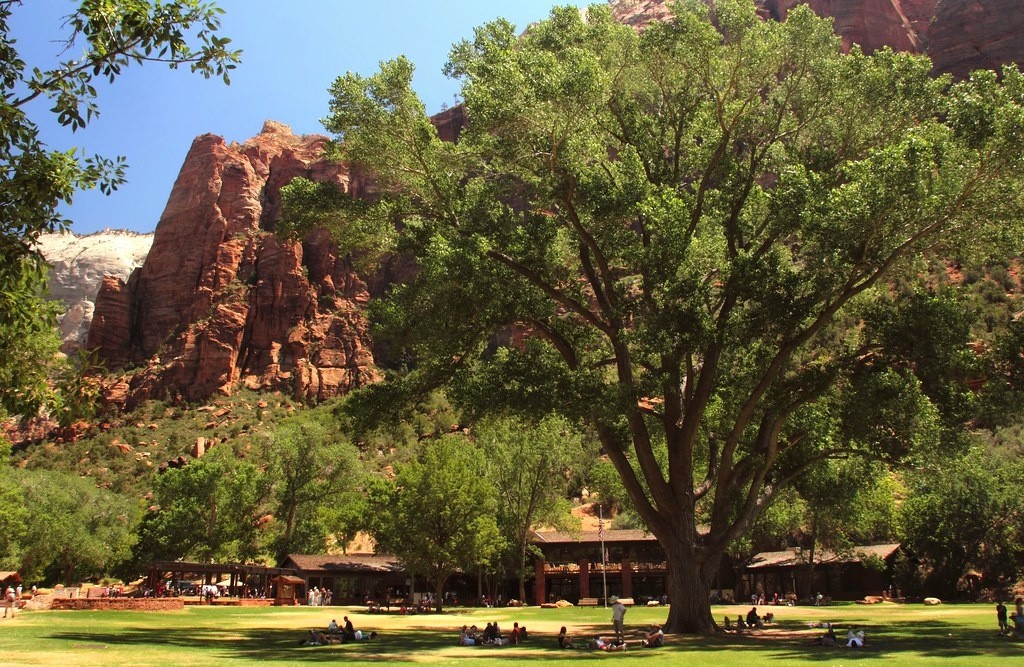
0;571;23;583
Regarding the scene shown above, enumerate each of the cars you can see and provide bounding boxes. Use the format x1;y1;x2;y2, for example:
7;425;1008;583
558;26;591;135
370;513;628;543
169;581;200;592
134;588;154;597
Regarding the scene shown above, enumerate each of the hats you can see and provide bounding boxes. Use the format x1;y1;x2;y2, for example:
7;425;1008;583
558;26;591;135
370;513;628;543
651;623;662;628
610;595;619;604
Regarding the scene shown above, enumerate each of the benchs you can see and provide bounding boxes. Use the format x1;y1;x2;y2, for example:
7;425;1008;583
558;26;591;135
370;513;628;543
390;598;406;607
212;597;239;605
180;596;206;605
617;599;635;608
578;598;598;608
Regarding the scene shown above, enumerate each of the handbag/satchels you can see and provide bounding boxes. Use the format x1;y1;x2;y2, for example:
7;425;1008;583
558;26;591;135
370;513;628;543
8;597;13;602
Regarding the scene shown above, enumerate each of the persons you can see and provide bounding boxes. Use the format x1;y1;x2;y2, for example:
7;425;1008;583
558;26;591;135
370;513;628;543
308;586;333;606
710;593;732;605
806;623;865;648
458;621;527;646
723;607;773;630
144;584;266;601
558;595;665;652
481;594;528;608
751;591;797;606
3;584;37;618
299;616;377;646
364;588;458;615
814;591;823;606
102;583;123;598
996;597;1024;636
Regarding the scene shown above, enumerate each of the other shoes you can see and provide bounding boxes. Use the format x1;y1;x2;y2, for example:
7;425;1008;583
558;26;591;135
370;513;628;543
624;643;627;651
608;643;612;652
11;615;14;618
2;616;6;618
